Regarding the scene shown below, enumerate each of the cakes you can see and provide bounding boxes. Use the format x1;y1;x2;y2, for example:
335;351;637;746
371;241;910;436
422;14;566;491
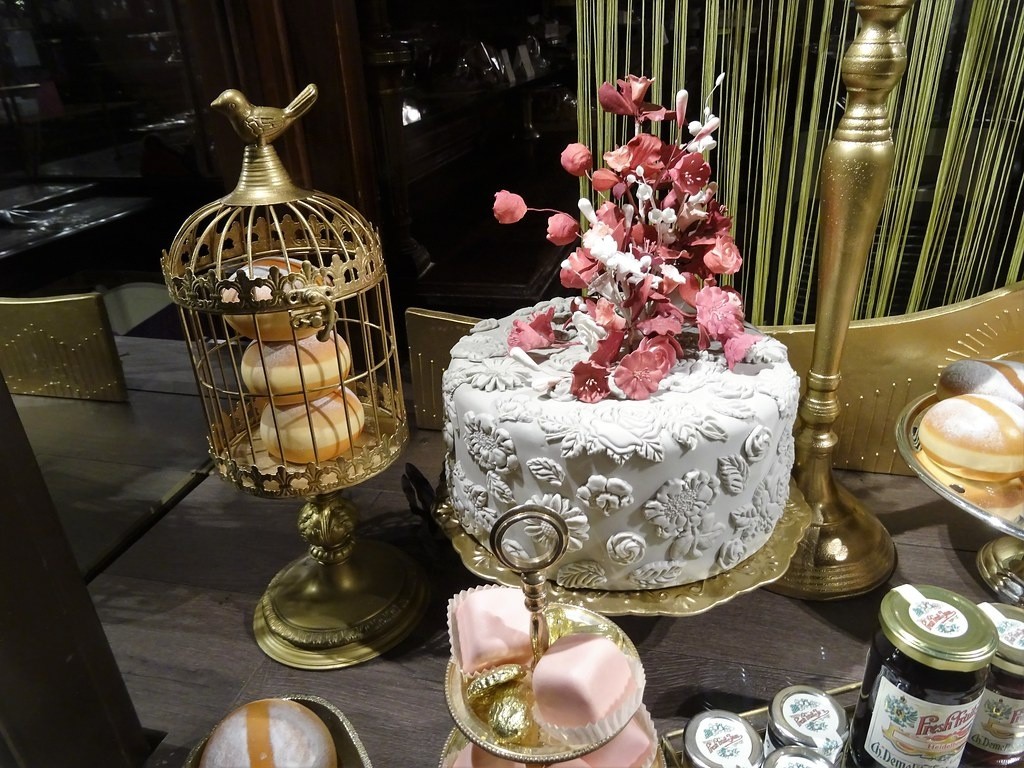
437;295;801;591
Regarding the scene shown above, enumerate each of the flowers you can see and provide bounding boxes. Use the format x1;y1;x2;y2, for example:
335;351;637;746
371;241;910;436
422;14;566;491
495;71;760;401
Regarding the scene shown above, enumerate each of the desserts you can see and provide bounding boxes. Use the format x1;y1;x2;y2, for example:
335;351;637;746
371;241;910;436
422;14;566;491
444;584;659;768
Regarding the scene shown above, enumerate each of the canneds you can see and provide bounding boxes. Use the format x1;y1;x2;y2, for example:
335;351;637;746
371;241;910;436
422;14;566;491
682;582;1024;768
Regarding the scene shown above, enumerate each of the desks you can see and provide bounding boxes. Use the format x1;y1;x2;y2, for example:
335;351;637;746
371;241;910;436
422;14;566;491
374;84;541;252
0;336;1024;768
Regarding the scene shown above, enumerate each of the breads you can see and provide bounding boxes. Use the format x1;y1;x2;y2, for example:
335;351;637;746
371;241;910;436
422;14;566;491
218;257;364;465
917;359;1024;483
199;697;338;768
